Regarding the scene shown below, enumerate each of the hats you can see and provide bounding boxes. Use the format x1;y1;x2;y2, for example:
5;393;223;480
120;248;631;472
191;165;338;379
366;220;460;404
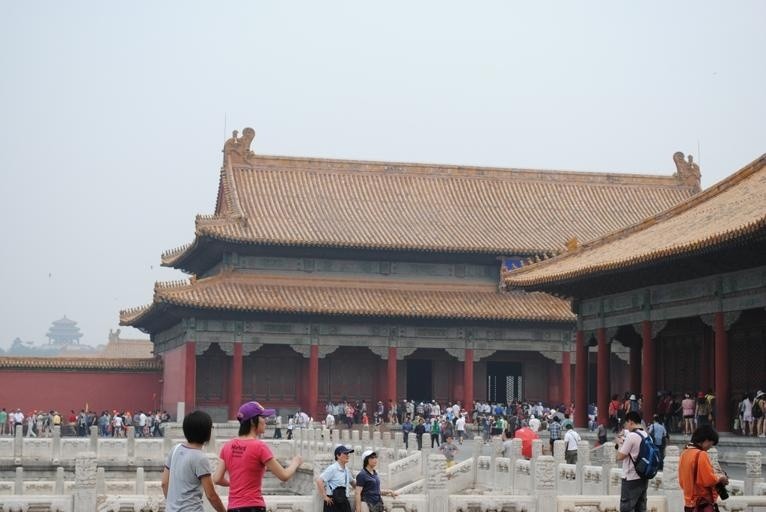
756;390;765;398
237;401;275;423
698;392;704;398
362;451;376;462
629;395;636;400
335;446;354;454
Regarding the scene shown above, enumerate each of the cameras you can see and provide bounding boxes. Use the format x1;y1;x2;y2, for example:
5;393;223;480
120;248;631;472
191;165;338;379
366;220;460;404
715;482;729;500
615;437;625;450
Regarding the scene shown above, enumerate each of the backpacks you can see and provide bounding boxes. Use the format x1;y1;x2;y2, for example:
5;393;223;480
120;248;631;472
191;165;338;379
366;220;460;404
752;399;762;418
628;432;661;479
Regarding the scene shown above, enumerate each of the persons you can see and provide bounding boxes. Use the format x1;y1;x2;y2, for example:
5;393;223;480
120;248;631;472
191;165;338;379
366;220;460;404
161;409;226;512
354;449;399;512
614;411;647;512
213;400;304;512
321;389;765;464
315;445;357;512
0;408;170;438
678;424;729;512
272;411;313;441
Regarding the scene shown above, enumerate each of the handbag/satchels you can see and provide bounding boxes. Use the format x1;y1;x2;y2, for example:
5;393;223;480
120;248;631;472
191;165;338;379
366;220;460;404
649;430;655;441
366;499;384;512
694;497;720;512
332;486;351;512
